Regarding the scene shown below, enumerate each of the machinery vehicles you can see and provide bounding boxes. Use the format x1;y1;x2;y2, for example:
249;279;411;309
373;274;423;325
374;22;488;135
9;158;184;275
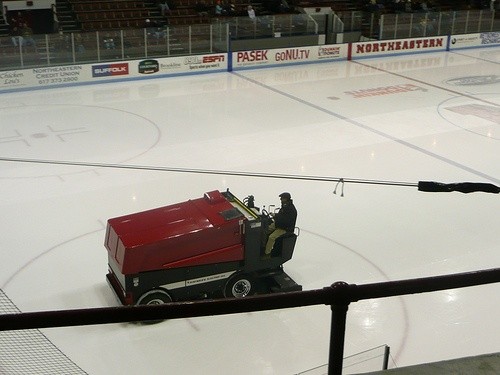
103;187;304;325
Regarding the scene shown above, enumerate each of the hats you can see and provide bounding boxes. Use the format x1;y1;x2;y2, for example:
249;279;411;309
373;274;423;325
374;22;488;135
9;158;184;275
278;192;290;197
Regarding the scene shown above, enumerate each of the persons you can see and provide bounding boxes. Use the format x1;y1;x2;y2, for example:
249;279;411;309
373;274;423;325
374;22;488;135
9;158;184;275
259;191;297;257
143;18;159;34
9;25;21;47
20;22;34;46
158;0;172;19
198;0;261;21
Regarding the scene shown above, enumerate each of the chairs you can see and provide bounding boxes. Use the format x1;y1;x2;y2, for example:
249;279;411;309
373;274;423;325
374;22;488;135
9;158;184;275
0;0;499;55
